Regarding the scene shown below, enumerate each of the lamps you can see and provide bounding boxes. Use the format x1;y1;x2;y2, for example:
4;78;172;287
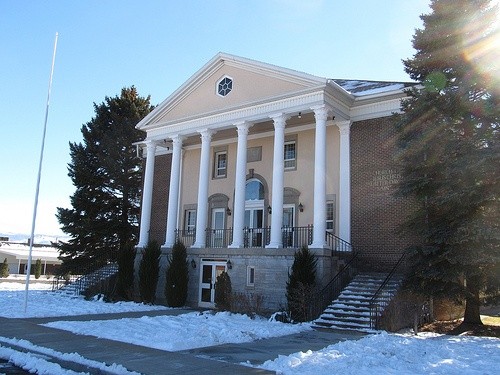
298;203;304;212
226;207;231;215
298;112;301;119
267;205;272;214
163;139;166;145
